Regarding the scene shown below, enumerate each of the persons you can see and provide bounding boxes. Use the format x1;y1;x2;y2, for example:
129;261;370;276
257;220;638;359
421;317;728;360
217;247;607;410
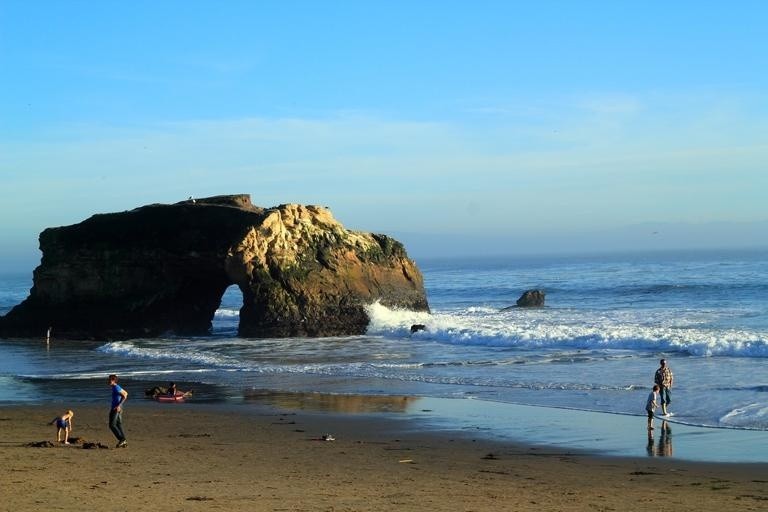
644;385;658;431
656;419;672;458
47;410;74;445
646;430;655;457
107;375;128;448
654;359;672;415
165;382;176;398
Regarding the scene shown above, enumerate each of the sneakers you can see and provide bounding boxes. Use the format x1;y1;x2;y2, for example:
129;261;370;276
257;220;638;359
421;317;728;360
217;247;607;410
116;440;128;448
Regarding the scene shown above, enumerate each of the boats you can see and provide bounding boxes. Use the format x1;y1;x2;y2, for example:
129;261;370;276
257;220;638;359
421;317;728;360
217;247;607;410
152;387;192;402
